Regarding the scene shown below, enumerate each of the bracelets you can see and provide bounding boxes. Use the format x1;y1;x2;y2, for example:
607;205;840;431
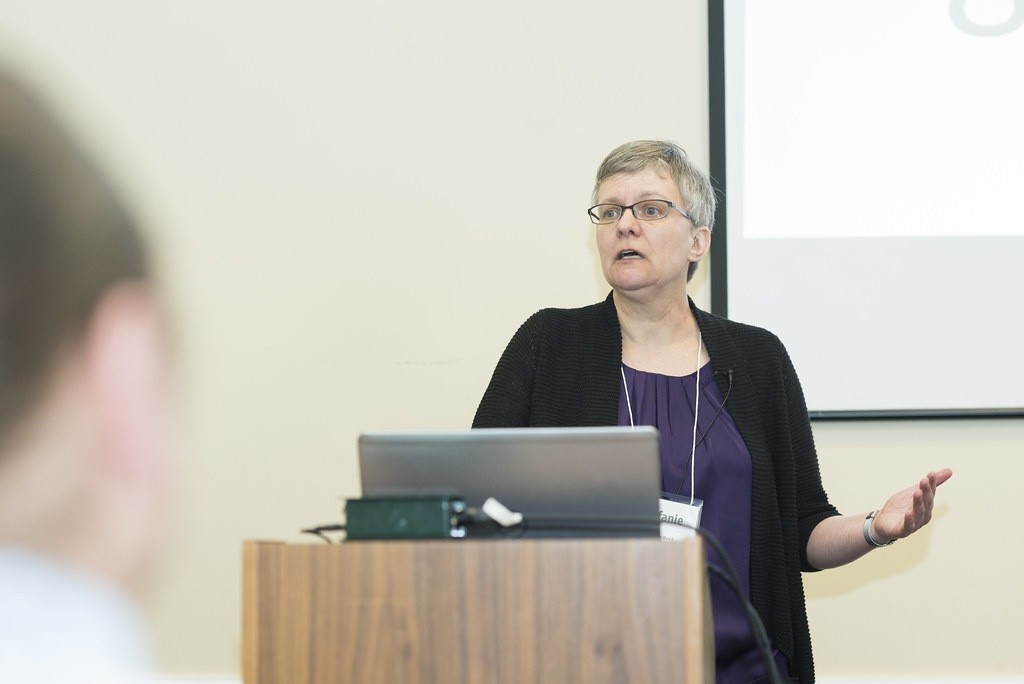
863;509;897;547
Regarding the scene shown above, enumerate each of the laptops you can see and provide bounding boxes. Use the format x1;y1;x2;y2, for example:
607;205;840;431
359;425;662;537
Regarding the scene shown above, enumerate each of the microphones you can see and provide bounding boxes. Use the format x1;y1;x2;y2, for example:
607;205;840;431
713;369;733;384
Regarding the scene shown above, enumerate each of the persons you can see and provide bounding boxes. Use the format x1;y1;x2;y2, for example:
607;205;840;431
472;140;952;684
0;63;172;684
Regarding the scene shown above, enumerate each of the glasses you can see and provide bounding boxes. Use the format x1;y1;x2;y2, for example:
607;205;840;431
588;199;689;225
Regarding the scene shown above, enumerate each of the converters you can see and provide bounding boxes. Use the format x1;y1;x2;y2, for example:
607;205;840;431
346;495;467;539
